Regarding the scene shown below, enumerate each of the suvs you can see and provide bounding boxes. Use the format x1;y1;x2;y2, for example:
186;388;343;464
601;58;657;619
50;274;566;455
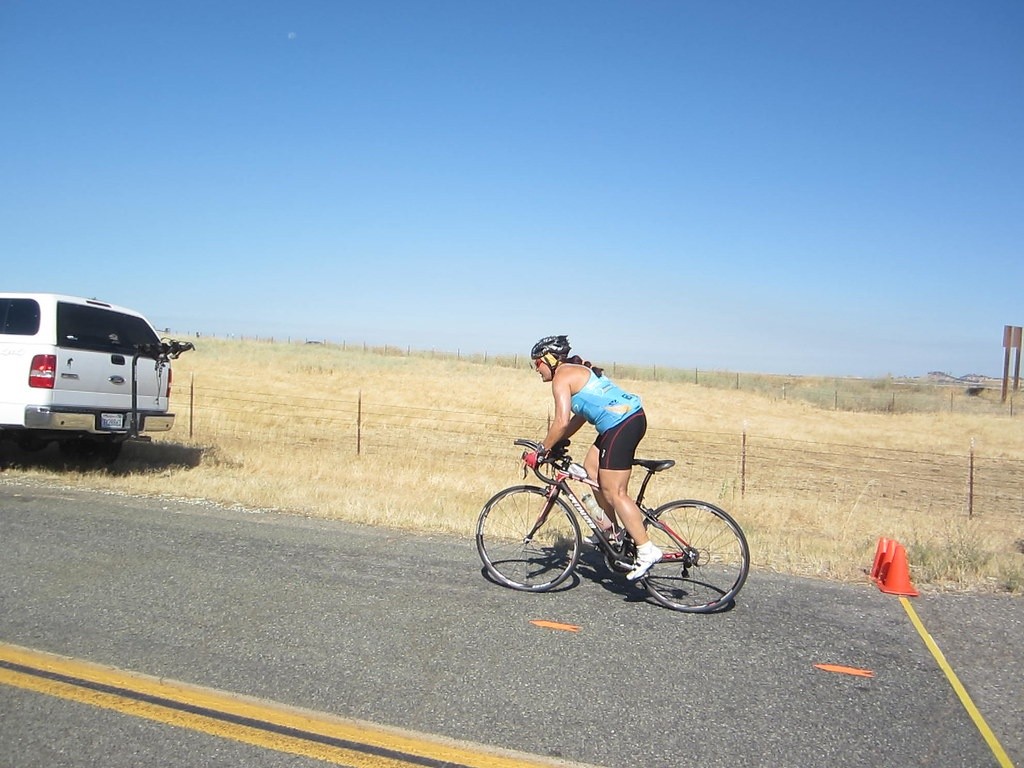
0;292;196;465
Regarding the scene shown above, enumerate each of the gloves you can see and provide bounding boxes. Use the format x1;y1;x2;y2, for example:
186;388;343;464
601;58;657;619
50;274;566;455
523;451;538;470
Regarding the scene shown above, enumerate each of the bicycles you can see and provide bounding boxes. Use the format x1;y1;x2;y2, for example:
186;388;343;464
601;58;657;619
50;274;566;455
475;439;749;613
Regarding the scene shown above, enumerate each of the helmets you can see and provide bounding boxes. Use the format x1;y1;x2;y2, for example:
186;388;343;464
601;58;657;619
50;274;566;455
530;335;572;360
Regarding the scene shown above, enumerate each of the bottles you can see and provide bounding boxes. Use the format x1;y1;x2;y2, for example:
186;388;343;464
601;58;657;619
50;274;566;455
581;493;612;531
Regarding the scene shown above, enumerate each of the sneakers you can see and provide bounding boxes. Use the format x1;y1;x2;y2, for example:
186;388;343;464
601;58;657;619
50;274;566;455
625;545;663;580
584;525;623;544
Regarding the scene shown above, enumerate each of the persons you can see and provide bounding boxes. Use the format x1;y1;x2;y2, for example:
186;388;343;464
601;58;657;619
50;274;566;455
523;335;664;579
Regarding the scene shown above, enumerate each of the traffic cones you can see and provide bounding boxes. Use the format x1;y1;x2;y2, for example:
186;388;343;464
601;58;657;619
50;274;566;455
877;539;898;583
878;545;919;595
873;537;888;579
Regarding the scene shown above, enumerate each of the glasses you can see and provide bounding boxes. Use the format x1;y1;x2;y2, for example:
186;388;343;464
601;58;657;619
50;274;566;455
534;359;543;368
